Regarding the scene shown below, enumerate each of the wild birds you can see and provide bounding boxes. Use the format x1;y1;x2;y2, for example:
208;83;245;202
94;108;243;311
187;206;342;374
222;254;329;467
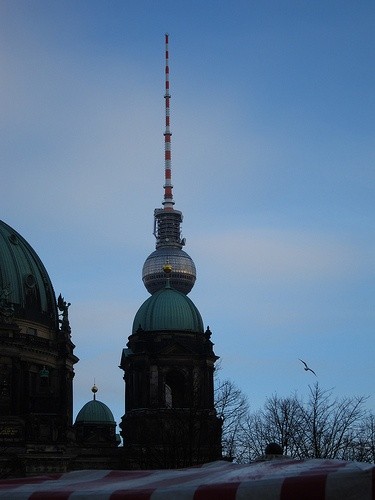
300;359;316;375
